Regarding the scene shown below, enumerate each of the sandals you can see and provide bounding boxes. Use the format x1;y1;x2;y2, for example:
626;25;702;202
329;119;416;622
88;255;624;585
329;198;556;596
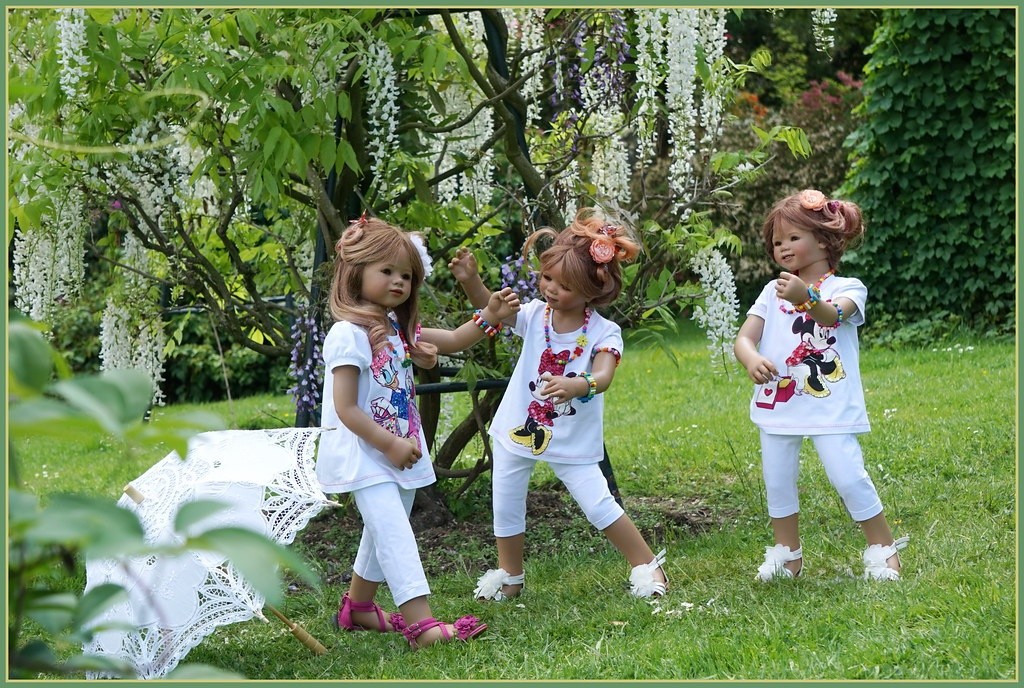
862;537;910;581
754;544;804;583
402;614;488;650
472;567;526;602
338;591;407;632
628;548;669;598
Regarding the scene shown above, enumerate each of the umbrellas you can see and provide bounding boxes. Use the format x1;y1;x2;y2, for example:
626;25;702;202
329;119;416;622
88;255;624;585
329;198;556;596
80;427;337;680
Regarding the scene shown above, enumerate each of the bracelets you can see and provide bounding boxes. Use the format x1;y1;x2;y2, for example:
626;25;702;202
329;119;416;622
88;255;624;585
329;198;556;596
576;371;597;403
473;309;503;336
792;284;821;314
415;322;421;343
817;299;844;330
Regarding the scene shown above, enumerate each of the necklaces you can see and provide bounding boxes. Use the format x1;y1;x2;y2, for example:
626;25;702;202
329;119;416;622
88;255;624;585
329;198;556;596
777;268;835;314
381;314;412;368
544;305;592;364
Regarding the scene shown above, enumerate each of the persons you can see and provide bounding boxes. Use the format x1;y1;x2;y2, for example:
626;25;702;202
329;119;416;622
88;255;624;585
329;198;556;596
315;209;521;652
733;189;910;584
448;207;671;608
